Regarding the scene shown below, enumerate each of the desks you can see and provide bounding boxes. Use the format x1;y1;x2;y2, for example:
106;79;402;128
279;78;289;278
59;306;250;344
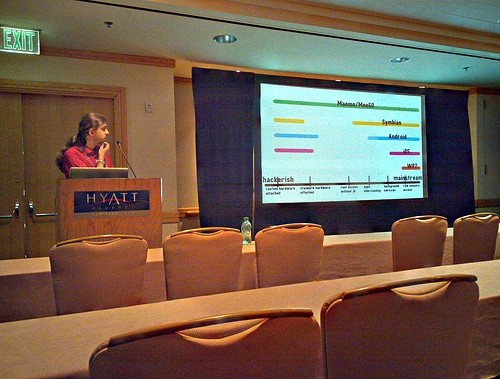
0;258;500;379
0;223;500;323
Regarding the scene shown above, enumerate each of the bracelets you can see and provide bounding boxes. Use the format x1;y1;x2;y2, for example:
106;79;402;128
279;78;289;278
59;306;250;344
96;159;106;166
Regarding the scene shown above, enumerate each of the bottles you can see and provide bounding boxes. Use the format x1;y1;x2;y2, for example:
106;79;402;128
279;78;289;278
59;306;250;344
241;217;252;246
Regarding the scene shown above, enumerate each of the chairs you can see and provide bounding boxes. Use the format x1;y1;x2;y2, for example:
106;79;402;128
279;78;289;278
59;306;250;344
49;212;500;379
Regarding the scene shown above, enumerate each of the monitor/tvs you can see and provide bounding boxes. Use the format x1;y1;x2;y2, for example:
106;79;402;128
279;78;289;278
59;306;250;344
68;166;129;178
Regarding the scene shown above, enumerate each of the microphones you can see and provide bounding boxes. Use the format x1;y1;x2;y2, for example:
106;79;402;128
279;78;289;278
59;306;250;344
117;139;137;177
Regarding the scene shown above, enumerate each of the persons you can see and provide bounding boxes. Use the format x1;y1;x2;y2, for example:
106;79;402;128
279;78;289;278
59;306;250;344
55;112;112;179
